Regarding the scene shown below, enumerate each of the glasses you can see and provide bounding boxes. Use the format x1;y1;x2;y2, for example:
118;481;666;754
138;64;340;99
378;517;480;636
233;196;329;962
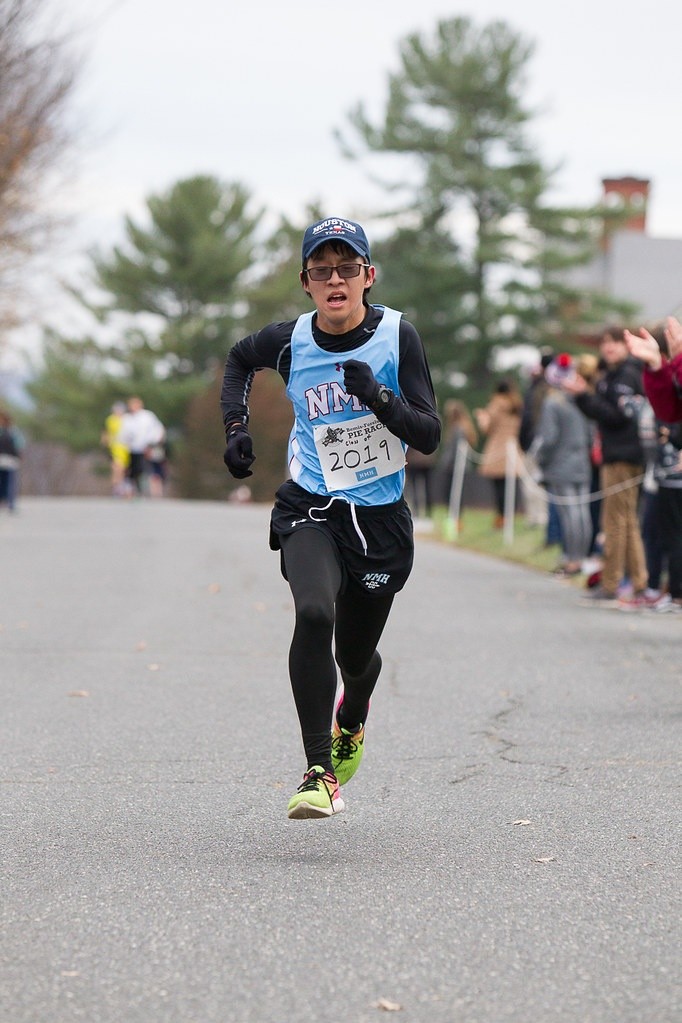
300;264;370;282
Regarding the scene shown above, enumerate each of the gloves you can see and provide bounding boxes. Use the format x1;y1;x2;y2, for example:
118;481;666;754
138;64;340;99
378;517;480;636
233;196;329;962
223;429;256;480
342;358;377;402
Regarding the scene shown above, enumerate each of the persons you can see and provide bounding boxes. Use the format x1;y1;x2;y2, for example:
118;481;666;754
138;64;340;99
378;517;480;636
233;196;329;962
0;411;23;514
219;217;440;822
103;400;133;499
120;397;167;500
440;314;681;617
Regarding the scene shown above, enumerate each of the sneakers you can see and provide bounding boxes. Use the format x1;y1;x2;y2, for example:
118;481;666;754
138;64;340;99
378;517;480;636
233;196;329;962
285;765;347;820
328;683;373;786
545;551;682;616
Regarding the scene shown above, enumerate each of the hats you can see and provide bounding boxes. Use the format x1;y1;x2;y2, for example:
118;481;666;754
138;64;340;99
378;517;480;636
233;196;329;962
299;217;370;266
